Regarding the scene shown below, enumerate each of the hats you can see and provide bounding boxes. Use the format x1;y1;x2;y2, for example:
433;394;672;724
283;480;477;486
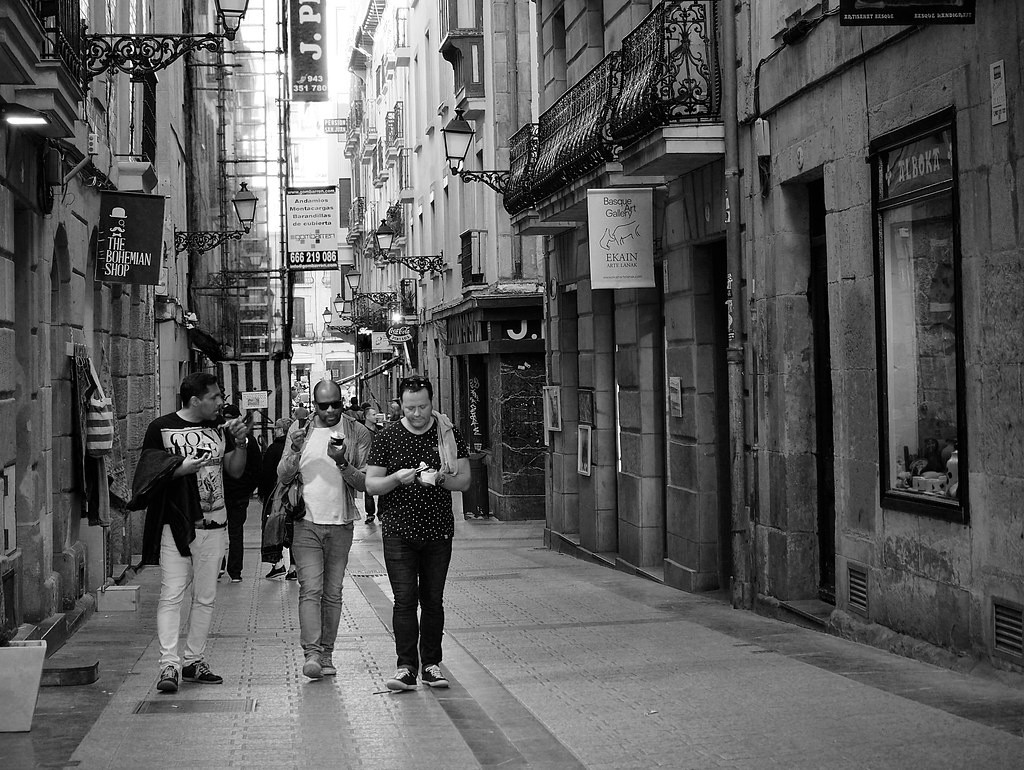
223;404;243;419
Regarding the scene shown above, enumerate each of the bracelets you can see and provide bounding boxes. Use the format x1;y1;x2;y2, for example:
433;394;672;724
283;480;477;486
234;438;248;449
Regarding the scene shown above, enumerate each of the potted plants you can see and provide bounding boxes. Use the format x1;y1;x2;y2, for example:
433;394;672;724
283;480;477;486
398;290;417;314
0;618;47;732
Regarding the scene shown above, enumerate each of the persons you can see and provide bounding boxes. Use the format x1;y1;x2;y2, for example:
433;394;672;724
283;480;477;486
293;402;309;420
217;404;262;582
366;376;471;690
347;395;384;525
258;417;297;581
132;372;248;691
276;379;370;679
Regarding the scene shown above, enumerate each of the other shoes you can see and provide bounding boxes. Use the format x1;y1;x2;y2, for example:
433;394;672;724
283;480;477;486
285;570;298;581
364;514;375;524
231;576;243;583
265;564;287;579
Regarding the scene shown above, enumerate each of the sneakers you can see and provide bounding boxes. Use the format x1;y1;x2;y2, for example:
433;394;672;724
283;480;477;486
155;665;179;692
302;653;325;679
421;664;449;687
387;667;418;690
320;655;337;675
181;658;223;685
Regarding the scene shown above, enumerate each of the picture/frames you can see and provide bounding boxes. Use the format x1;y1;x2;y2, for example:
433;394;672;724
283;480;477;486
576;389;597;429
577;425;591;476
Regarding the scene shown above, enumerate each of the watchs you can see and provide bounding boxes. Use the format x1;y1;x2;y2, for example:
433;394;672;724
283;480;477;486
438;474;445;486
336;460;348;470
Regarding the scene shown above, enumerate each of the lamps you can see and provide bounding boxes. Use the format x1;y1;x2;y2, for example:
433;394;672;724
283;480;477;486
262;309;283;333
174;182;259;262
262;288;275;305
335;294;360;325
3;103;53;126
441;106;511;194
77;0;250;96
247;241;266;265
346;265;395;307
373;218;443;276
323;307;358;335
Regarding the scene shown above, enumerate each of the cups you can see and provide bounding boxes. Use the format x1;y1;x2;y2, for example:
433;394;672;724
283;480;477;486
196;447;212;461
330;431;346;452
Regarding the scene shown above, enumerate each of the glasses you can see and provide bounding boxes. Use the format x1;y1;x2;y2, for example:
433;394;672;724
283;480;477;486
315;400;342;410
399;374;428;387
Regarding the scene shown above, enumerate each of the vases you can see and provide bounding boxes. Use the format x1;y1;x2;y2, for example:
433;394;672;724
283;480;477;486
946;451;958;491
